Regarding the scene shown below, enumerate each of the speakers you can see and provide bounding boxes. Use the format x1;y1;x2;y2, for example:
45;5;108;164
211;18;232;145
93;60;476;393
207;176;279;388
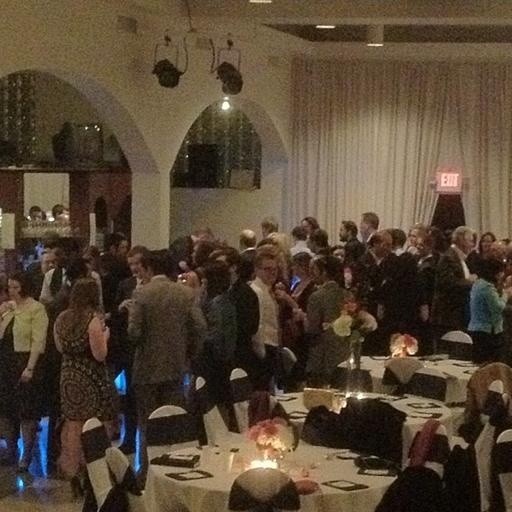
188;143;222;188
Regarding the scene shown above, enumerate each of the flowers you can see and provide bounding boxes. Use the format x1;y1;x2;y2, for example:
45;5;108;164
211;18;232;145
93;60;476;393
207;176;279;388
322;296;380;342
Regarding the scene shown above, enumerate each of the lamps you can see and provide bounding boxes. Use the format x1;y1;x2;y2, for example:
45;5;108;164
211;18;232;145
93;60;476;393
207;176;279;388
363;22;386;47
152;0;243;96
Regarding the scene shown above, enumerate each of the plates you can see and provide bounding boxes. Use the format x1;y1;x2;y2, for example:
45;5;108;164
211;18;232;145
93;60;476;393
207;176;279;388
28;135;44;162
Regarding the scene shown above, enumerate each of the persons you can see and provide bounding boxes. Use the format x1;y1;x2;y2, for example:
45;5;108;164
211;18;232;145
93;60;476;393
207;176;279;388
1;205;512;497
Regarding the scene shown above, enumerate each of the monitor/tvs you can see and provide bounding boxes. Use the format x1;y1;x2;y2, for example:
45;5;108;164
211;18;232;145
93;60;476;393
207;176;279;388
53;122;104;168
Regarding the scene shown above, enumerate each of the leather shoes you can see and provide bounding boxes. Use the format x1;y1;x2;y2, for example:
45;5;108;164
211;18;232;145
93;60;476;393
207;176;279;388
18;453;32;473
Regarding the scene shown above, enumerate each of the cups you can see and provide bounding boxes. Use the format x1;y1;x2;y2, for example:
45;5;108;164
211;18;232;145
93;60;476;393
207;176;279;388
227;453;244;475
